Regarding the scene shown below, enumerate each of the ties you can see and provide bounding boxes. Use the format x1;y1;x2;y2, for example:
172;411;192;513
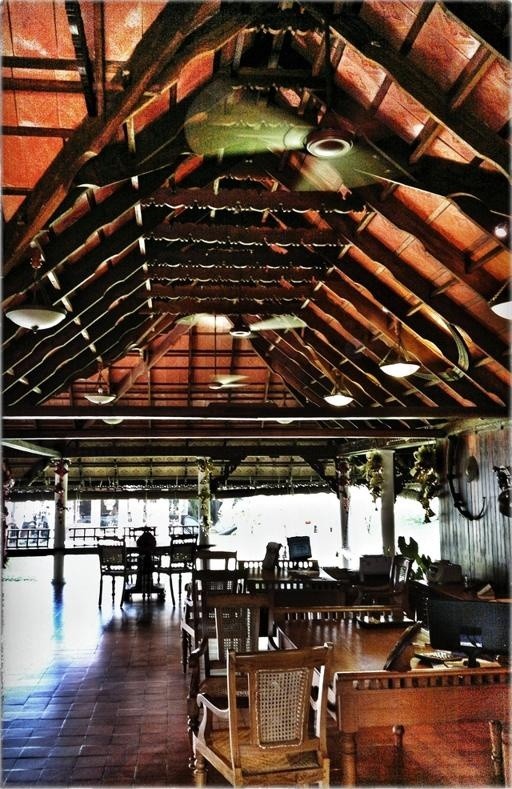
427;599;512;669
287;536;312;570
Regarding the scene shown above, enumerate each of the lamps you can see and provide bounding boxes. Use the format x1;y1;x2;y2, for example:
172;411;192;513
84;371;116;405
487;275;512;320
378;323;421;378
4;248;68;333
104;419;124;426
324;379;354;407
277;397;292;424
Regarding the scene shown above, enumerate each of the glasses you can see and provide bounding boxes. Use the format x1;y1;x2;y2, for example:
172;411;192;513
84;371;116;405
4;248;68;333
104;419;124;426
324;379;354;407
487;275;512;320
378;323;421;378
277;397;292;424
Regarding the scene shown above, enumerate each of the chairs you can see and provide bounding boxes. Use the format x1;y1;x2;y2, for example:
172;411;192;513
180;535;511;787
96;527;236;613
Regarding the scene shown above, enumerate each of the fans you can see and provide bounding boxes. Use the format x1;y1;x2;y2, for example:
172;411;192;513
185;52;387;192
174;303;306;338
195;370;249;391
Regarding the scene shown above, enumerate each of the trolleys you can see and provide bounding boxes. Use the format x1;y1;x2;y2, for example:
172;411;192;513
195;370;249;391
185;52;387;192
174;303;306;338
